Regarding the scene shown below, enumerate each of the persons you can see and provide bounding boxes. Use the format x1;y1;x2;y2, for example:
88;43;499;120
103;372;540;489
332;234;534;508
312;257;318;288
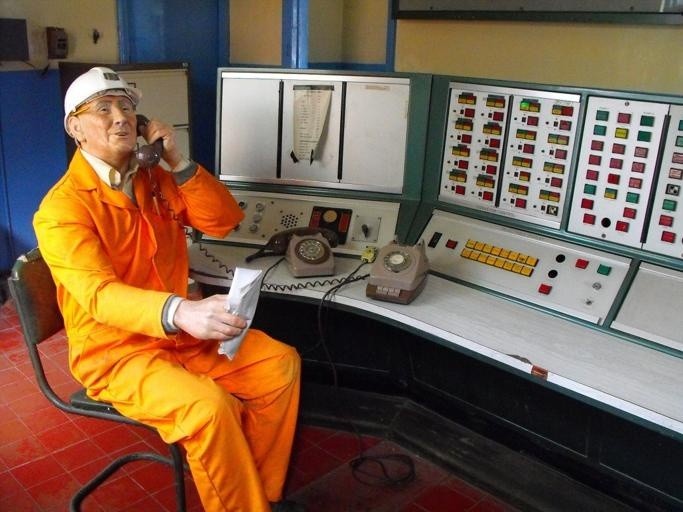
31;65;305;512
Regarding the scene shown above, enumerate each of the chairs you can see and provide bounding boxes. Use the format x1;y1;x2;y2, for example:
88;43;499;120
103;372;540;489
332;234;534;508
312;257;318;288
7;247;194;512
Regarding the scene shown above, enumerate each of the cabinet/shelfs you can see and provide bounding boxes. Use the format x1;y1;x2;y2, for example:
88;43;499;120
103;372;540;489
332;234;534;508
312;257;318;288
57;57;196;242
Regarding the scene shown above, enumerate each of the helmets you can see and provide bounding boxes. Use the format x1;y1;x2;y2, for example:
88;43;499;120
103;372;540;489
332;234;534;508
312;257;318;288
62;64;144;139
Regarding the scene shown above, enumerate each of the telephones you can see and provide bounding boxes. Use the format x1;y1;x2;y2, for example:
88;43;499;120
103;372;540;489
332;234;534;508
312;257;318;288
136;114;163;169
270;227;338;278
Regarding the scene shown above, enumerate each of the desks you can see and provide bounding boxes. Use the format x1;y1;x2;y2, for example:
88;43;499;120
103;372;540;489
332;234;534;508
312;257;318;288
178;67;681;510
